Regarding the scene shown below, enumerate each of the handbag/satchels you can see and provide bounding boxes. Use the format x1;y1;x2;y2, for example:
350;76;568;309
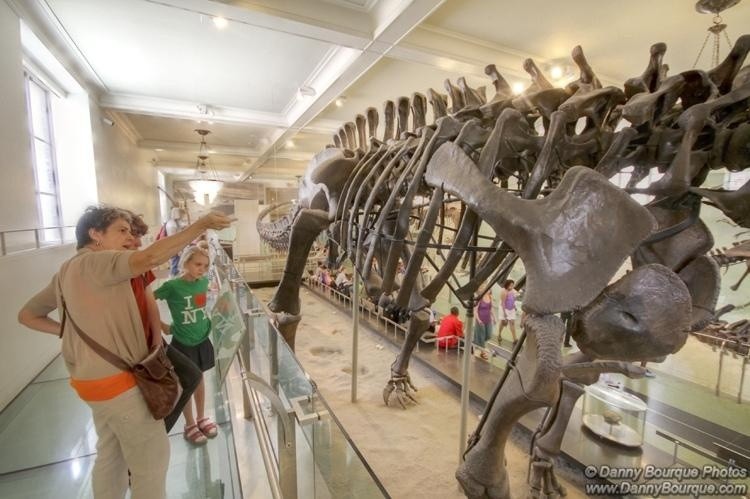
130;346;179;418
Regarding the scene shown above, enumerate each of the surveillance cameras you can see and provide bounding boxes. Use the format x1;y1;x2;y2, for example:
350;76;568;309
103;118;114;126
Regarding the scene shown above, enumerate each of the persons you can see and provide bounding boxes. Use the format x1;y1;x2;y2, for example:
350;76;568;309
561;312;572;347
306;261;518;360
18;203;239;499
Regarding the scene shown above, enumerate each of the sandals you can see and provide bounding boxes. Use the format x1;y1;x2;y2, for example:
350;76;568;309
479;353;488;359
183;425;206;445
196;417;218;437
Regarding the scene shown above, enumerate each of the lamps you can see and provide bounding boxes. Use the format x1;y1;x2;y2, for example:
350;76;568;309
188;127;224;206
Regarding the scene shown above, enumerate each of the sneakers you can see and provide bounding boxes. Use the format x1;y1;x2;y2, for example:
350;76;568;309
564;344;572;347
497;336;518;344
